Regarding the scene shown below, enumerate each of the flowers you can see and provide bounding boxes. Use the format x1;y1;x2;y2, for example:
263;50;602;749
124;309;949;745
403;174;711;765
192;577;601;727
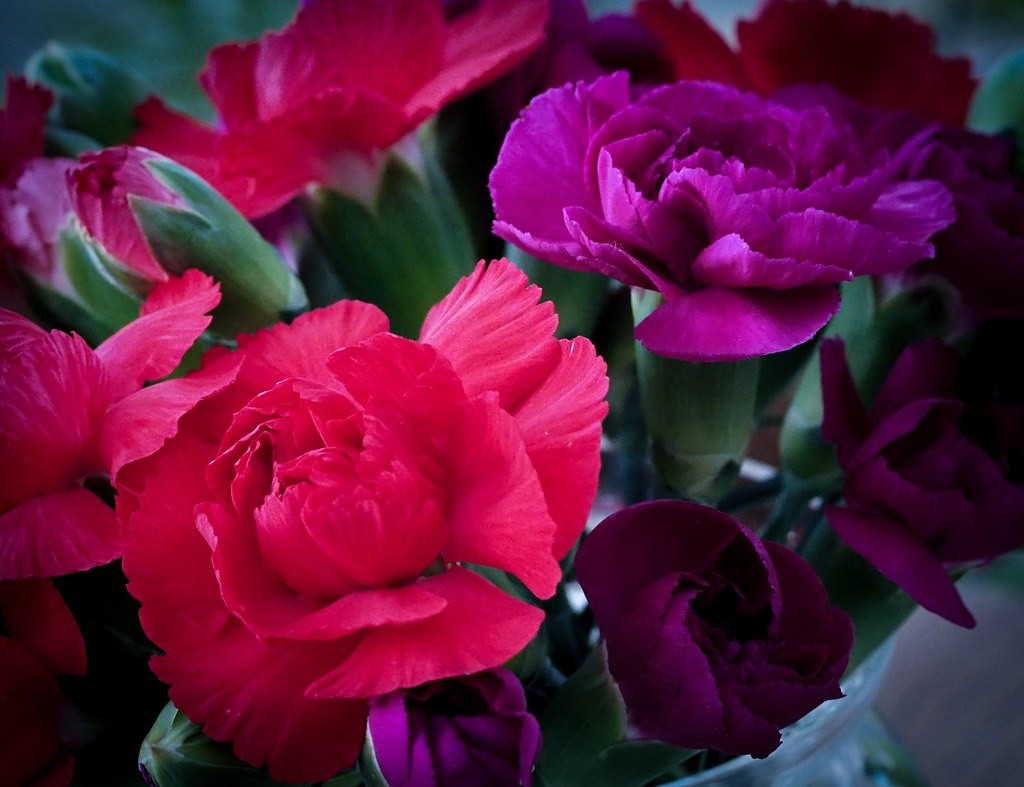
0;0;1024;787
